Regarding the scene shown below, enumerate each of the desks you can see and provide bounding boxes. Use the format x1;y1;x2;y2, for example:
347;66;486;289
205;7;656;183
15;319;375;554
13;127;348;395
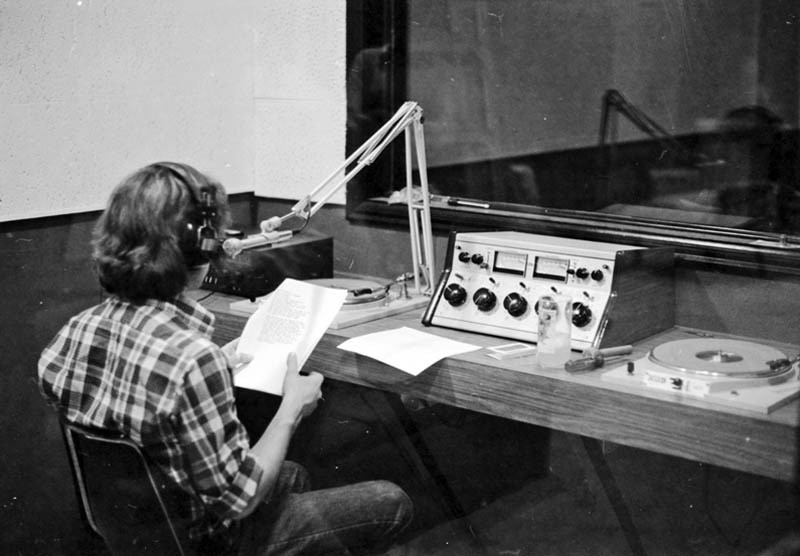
183;263;799;556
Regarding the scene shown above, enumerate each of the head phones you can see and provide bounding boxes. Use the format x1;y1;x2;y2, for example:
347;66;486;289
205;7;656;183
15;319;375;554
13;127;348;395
148;162;219;271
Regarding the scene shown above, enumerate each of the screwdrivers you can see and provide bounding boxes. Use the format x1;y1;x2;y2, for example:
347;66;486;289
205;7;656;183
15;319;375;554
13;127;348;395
429;194;489;209
565;354;622;373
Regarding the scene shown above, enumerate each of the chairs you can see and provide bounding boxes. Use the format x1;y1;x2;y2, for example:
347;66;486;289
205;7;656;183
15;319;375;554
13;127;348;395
57;416;192;556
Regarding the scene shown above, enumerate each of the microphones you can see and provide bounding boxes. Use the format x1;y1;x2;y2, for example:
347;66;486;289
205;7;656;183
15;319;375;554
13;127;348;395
221;229;298;258
694;159;729;169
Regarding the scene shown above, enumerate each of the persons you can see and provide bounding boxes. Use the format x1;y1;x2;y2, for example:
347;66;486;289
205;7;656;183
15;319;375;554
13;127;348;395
36;161;416;556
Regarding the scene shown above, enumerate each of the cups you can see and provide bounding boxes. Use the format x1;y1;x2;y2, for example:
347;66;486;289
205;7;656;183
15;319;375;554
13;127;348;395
538;294;573;373
